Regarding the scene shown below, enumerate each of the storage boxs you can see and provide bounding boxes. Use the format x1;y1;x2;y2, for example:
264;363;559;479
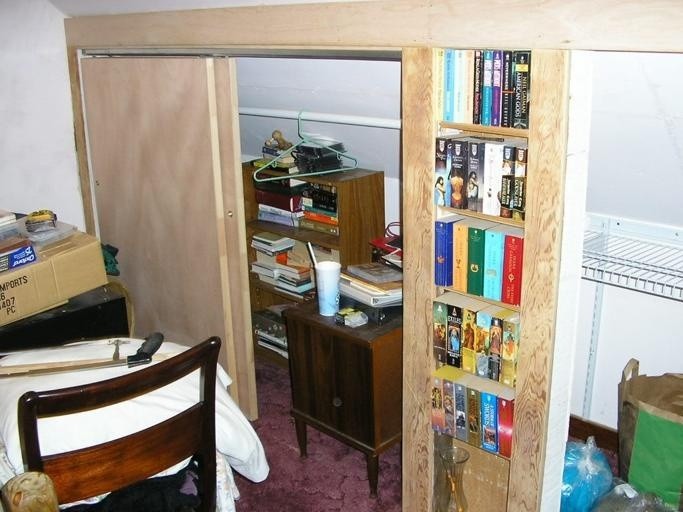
1;210;105;326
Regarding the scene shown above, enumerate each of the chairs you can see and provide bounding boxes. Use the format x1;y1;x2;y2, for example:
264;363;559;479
15;335;222;510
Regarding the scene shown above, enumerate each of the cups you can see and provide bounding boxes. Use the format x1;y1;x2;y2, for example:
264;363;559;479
314;262;342;318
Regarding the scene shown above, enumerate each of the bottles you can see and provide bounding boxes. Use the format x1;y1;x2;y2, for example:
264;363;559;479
437;446;471;512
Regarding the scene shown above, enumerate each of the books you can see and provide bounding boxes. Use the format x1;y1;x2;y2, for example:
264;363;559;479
438;48;529;129
430;364;516;457
435;133;525;221
434;215;525;306
0;219;16;228
432;289;520;387
248;142;404;361
0;211;16;223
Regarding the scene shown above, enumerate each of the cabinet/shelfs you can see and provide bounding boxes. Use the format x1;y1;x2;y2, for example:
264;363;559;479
402;50;569;511
242;165;384;370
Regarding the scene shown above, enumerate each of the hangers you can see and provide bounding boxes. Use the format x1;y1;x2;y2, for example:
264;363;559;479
255;112;358;183
